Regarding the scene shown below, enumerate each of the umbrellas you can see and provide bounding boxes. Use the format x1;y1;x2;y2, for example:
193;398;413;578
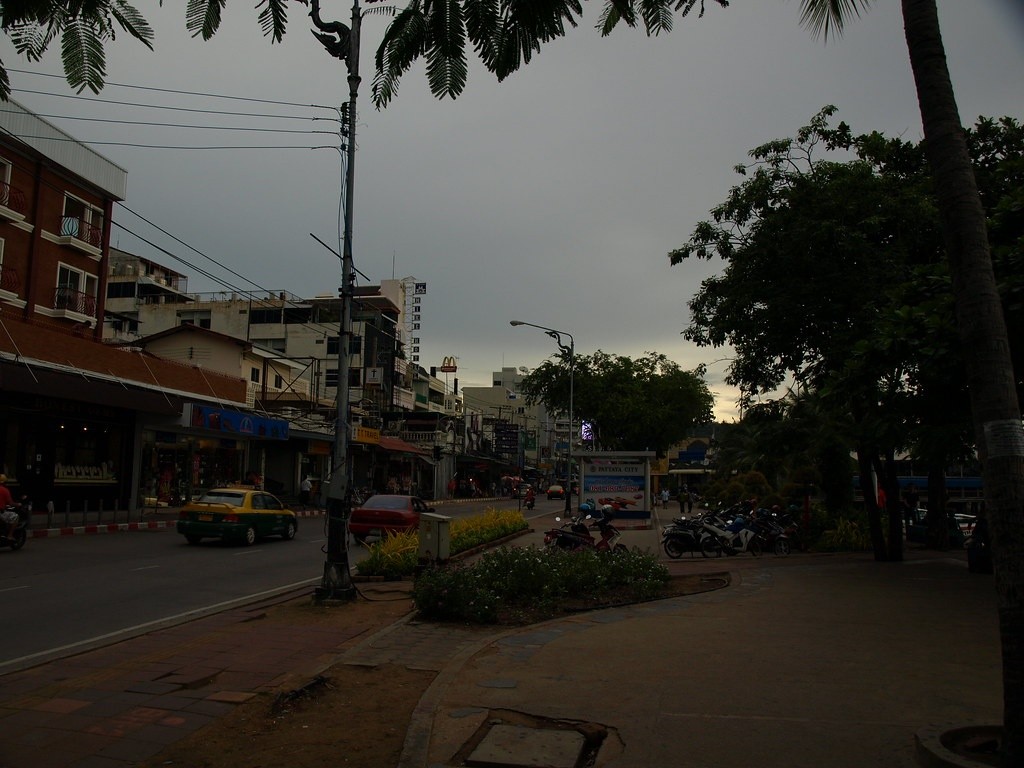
502;475;525;482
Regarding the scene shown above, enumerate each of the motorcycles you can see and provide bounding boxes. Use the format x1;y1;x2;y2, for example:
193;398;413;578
527;498;535;510
544;504;629;552
544;516;590;547
660;500;800;559
576;488;578;496
0;501;28;549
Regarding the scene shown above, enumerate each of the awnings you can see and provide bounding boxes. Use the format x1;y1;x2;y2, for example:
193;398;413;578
378;438;425;454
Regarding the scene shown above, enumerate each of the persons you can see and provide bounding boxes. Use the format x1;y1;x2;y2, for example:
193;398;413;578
0;474;22;541
302;475;313;510
864;480;921;545
522;484;535;507
660;481;695;513
447;476;523;500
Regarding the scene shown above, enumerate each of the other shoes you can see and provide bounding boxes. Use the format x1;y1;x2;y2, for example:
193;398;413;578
7;536;16;541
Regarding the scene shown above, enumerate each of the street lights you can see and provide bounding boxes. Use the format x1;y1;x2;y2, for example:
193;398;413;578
510;320;574;518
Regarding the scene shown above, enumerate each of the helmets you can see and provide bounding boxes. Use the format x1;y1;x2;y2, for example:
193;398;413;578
601;505;612;514
0;474;8;483
772;505;780;510
734;518;744;525
580;503;591;510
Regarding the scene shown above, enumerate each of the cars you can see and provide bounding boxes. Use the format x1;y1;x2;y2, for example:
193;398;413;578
348;495;436;546
516;483;533;498
176;488;298;545
902;508;984;547
546;485;565;500
